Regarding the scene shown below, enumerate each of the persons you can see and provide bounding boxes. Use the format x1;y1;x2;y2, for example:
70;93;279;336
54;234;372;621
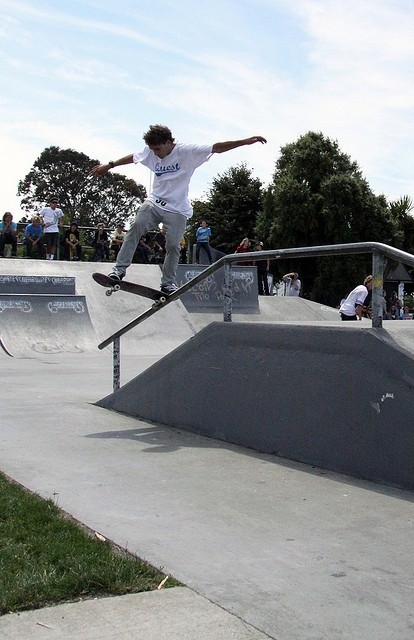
64;222;87;262
38;197;63;260
0;212;17;258
382;289;414;320
89;124;268;294
195;221;215;265
22;220;46;259
133;229;187;264
272;272;301;297
336;275;373;321
253;241;268;294
92;224;109;262
234;238;255;265
109;223;124;262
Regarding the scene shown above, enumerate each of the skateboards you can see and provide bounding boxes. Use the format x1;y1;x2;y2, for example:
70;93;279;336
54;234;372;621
91;273;170;310
69;245;78;261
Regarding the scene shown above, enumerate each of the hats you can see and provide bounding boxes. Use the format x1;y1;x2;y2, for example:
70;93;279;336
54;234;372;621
256;241;264;246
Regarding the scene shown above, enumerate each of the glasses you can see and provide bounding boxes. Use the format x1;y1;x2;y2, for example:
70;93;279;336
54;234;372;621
52;202;58;204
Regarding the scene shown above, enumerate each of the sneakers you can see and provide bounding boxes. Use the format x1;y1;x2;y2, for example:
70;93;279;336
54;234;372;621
161;283;179;292
51;255;54;260
46;254;50;259
108;272;125;281
